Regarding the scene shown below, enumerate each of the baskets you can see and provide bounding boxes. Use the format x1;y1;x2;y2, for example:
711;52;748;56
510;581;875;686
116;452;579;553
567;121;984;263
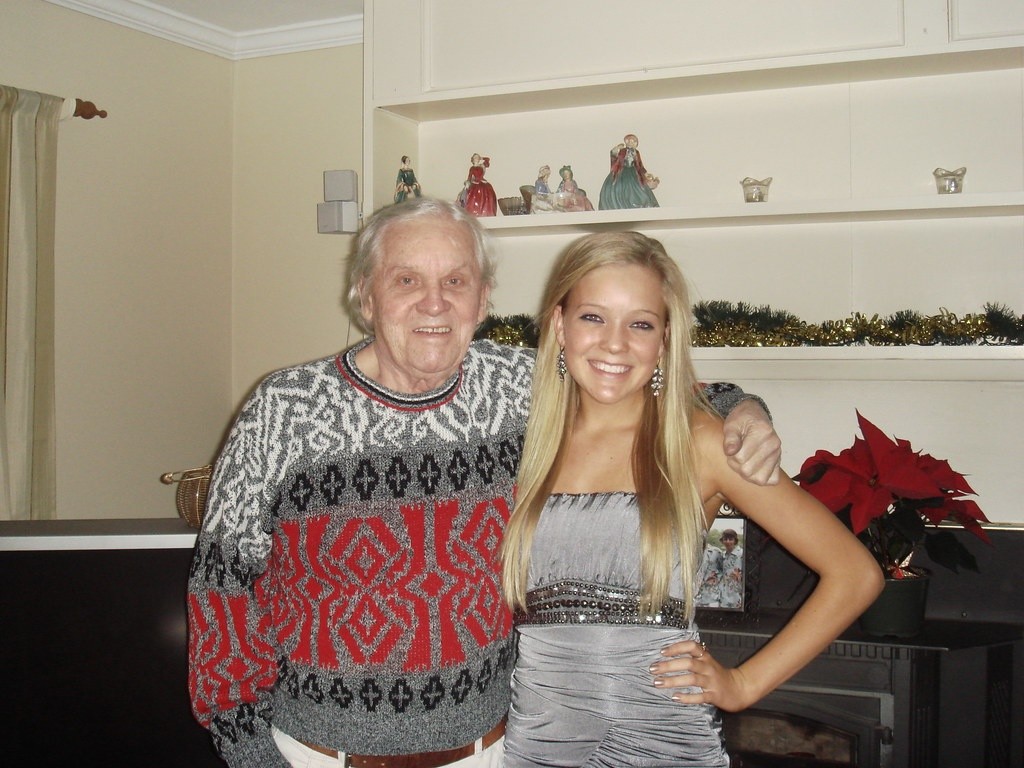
160;465;214;528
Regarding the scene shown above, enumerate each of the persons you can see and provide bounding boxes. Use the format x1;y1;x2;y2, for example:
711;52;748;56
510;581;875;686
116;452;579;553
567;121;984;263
500;230;885;768
392;133;663;209
693;529;744;608
188;199;782;768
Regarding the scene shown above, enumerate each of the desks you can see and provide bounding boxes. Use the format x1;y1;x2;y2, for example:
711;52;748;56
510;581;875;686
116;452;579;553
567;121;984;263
691;605;1024;768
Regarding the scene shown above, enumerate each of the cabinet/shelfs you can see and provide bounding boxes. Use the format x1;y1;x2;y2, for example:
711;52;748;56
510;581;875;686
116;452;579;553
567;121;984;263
364;0;1024;363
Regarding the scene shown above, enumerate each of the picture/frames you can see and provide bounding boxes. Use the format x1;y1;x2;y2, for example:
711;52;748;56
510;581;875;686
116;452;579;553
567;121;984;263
695;500;762;632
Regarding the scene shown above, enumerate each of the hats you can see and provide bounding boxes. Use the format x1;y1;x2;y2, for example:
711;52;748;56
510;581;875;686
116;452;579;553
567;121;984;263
720;530;739;546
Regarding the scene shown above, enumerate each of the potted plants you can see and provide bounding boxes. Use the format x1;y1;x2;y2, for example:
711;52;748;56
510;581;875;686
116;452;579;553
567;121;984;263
783;404;994;641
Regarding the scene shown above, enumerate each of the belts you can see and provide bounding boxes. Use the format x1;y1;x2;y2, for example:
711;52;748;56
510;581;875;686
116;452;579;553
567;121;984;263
295;710;507;768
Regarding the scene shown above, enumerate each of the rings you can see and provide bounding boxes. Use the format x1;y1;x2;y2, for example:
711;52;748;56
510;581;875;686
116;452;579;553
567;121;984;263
693;641;708;658
